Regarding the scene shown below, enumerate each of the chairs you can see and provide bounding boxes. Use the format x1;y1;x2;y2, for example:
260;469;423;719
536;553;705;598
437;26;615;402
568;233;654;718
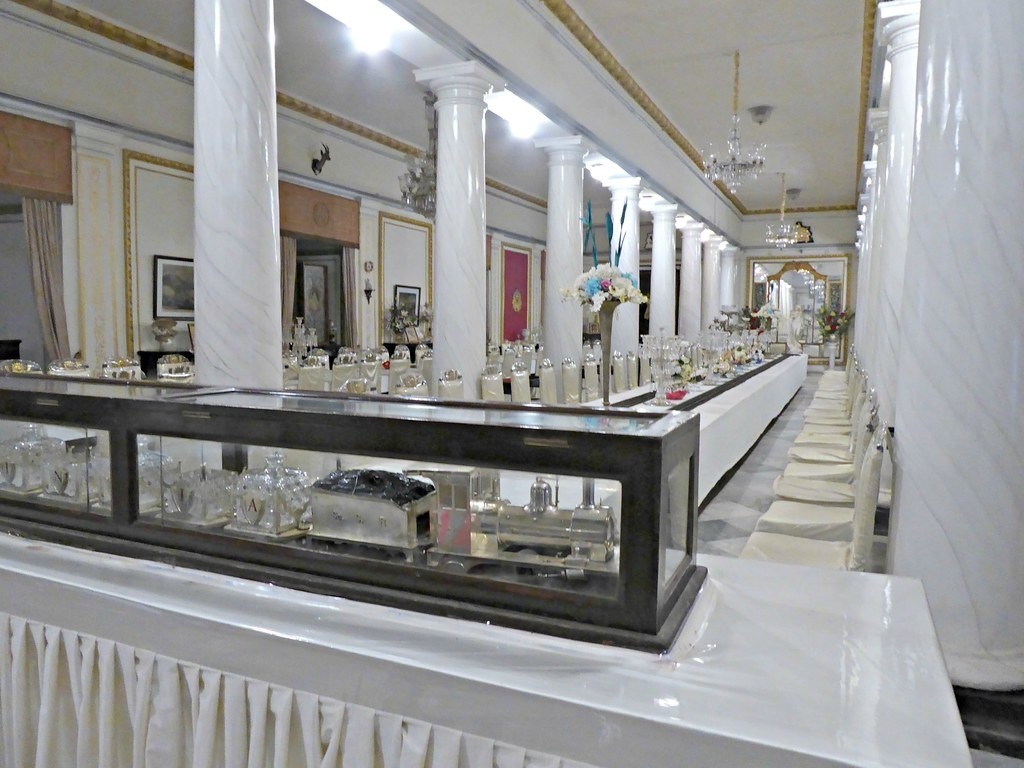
0;341;787;402
741;343;890;571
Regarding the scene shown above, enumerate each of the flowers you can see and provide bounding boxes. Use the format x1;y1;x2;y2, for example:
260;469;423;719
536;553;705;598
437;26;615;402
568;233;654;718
386;301;418;334
558;193;649;313
818;304;855;342
671;301;781;382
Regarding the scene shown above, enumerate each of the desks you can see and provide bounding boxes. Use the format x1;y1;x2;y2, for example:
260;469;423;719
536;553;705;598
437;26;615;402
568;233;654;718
505;352;809;534
0;533;972;768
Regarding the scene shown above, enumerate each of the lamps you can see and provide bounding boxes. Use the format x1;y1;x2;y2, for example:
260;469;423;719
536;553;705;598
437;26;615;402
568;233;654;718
748;105;774;126
786;189;801;200
765;172;799;250
701;48;768;194
398;91;438;219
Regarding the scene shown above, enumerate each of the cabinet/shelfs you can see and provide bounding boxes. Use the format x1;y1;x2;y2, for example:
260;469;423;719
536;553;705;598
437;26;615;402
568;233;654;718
0;369;709;653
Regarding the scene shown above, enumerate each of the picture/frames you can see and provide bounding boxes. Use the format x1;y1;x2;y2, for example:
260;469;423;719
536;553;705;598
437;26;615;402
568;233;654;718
394;284;421;326
153;255;194;320
499;241;533;346
377;210;434;348
122;149;194;358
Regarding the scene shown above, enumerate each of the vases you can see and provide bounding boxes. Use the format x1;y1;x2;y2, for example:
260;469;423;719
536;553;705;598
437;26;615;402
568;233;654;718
394;332;405;344
829;334;836;343
